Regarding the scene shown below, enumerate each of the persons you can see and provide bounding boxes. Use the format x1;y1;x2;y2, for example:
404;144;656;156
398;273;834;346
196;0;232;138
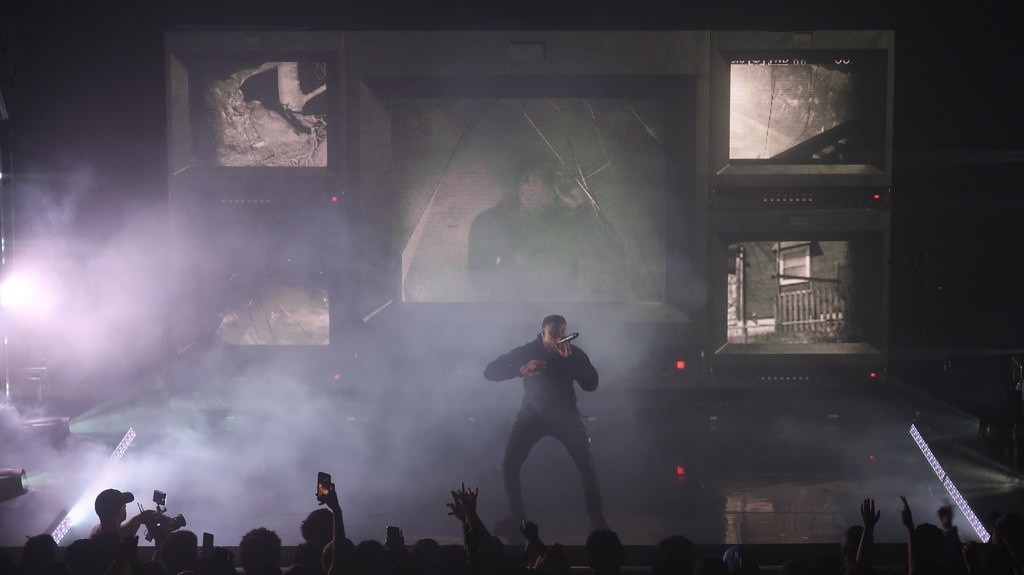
1;471;1024;575
482;314;615;534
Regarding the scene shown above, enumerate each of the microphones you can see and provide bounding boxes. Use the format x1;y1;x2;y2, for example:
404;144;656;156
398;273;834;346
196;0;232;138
557;332;579;345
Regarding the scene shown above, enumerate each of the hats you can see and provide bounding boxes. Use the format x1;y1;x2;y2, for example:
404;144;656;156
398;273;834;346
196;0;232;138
992;513;1024;545
95;489;134;513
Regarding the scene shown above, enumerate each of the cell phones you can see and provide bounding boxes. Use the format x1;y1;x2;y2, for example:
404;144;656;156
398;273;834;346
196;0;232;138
202;533;213;549
317;472;331;501
386;526;400;546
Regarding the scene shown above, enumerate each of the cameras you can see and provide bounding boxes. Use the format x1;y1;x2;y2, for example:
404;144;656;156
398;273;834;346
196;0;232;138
145;491;185;543
551;543;564;552
